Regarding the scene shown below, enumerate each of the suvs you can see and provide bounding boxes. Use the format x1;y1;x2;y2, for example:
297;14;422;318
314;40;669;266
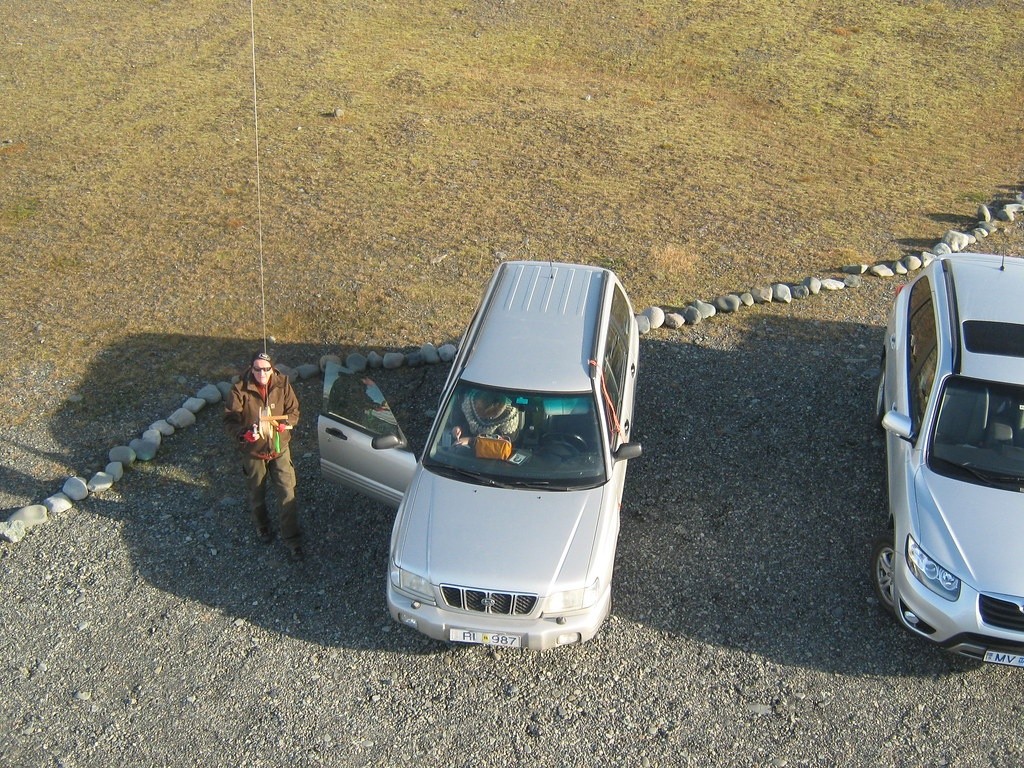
317;260;647;655
872;254;1024;670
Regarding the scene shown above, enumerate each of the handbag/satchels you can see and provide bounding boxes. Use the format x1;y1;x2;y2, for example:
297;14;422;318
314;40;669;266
475;432;512;460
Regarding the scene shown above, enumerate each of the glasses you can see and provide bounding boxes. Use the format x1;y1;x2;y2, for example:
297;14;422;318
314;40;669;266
254;367;272;371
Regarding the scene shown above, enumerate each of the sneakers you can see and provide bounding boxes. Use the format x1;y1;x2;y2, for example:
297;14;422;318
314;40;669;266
257;527;272;543
291;545;305;561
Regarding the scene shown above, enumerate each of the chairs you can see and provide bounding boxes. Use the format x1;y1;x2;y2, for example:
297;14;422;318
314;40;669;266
935;381;990;446
543;404;597;452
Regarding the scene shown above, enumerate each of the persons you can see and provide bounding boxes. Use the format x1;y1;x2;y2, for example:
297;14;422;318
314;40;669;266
224;349;306;560
327;372;390;430
450;387;520;449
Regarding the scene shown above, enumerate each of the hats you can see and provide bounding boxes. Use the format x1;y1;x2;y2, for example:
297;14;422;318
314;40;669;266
251;353;272;367
